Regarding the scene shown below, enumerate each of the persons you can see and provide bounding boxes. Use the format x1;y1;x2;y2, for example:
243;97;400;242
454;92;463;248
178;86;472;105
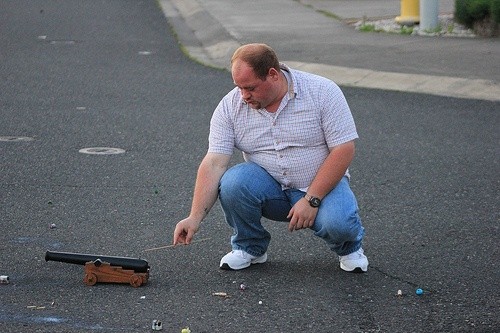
171;43;373;273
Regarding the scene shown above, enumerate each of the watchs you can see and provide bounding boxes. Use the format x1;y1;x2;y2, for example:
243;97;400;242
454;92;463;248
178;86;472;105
303;192;322;209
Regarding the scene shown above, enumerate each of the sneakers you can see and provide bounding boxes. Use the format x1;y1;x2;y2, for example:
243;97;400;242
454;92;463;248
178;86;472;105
218;249;269;270
337;249;370;275
